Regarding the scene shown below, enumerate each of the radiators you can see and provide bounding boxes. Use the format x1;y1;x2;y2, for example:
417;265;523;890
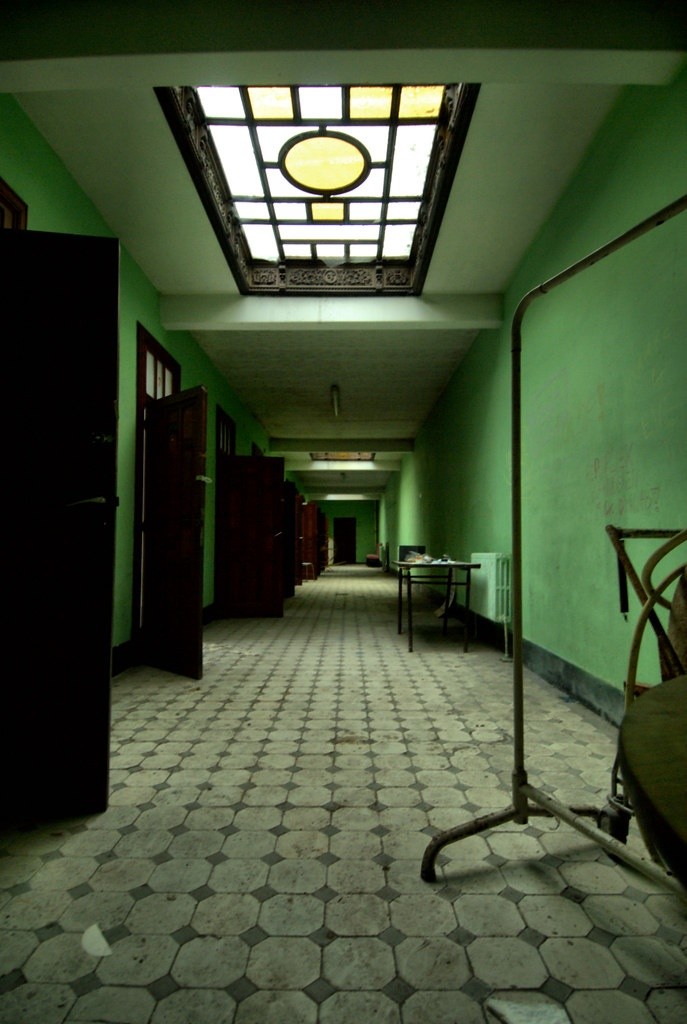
469;551;511;621
379;546;383;561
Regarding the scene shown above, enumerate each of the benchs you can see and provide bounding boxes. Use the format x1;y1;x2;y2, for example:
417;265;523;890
366;555;379;567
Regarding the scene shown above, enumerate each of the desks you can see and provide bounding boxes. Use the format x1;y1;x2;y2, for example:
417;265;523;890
389;559;481;653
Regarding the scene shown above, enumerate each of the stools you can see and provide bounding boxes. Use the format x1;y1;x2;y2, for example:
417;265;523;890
303;562;314;578
